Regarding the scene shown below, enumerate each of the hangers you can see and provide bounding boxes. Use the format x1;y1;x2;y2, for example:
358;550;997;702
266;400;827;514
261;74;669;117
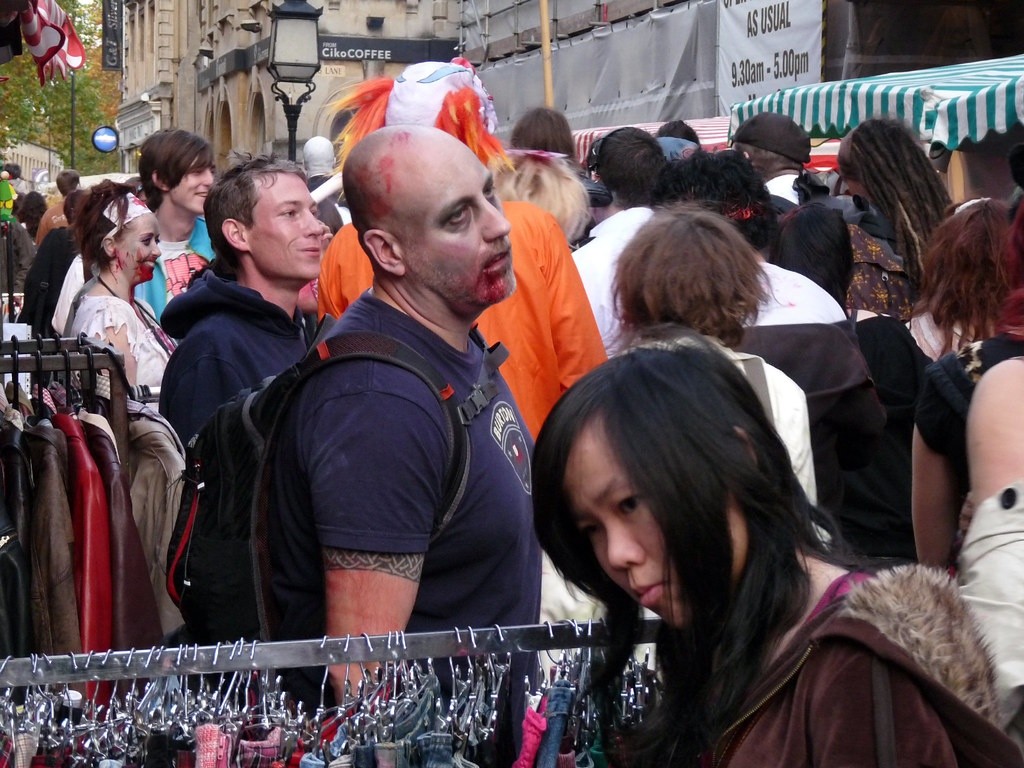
10;332;100;423
0;618;660;768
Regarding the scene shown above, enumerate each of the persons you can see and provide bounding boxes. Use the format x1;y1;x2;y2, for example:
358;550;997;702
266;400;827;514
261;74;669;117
1;58;1024;768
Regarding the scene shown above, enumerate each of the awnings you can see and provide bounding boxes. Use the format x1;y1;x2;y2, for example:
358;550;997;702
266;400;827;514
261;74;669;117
0;0;85;85
568;115;730;169
728;53;1024;153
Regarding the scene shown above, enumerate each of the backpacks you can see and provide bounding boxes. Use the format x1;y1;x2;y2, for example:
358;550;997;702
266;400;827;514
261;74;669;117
165;312;485;722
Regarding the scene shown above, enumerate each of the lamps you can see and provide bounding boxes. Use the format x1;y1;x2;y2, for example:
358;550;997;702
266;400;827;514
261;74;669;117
240;20;261;32
367;17;385;26
199;46;214;59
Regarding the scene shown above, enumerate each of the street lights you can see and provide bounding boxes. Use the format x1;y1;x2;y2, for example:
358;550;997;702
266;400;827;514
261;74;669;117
266;0;323;162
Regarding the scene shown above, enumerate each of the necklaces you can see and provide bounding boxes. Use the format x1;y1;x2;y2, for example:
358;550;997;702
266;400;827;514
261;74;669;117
97;275;178;357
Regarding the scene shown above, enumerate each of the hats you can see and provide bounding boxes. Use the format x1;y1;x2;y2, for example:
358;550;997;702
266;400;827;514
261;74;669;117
587;139;605;170
730;113;812;162
